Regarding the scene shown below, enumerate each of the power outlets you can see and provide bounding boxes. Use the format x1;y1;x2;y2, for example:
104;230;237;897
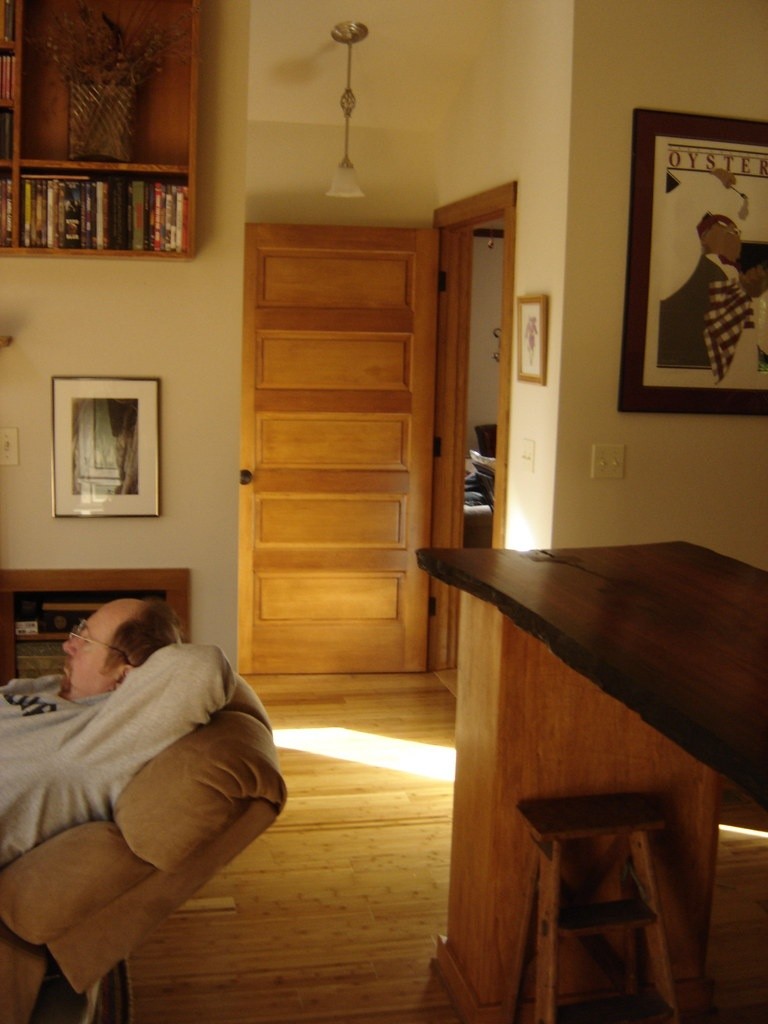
0;427;20;466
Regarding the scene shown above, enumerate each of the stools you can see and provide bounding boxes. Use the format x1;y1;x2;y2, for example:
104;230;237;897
514;789;684;1024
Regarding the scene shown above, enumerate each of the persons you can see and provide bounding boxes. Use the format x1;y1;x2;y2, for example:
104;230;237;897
0;599;234;867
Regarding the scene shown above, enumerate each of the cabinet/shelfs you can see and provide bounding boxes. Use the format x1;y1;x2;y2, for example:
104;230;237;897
0;0;201;259
0;569;192;687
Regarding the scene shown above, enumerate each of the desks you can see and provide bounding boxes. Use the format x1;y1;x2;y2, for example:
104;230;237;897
416;539;768;1024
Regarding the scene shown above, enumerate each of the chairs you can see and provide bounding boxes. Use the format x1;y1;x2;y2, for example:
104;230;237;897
475;424;496;493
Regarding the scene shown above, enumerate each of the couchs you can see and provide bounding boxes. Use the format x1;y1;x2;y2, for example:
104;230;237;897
0;671;288;1024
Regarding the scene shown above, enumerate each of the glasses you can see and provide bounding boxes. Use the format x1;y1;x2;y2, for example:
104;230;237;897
70;620;130;665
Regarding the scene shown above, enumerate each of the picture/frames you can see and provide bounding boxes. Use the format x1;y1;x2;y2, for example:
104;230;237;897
516;295;548;386
51;375;161;519
616;110;768;417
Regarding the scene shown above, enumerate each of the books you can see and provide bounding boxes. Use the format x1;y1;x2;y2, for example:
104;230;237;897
0;175;188;253
0;55;16;99
0;0;15;42
0;109;13;159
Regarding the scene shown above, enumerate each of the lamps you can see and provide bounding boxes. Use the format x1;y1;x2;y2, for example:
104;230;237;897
325;20;370;198
